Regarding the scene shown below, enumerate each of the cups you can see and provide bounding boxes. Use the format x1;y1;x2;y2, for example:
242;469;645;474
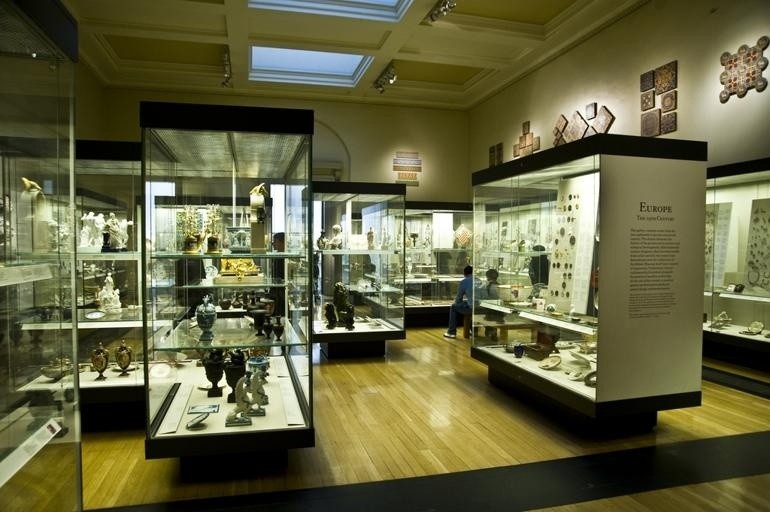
514;345;526;359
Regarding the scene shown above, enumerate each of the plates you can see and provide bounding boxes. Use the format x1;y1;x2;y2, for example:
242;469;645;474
538;355;562;370
84;310;106;319
510;301;533;307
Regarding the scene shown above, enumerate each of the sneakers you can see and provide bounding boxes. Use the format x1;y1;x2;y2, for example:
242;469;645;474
443;329;497;339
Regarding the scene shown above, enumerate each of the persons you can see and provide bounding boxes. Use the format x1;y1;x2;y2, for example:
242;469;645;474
174;236;208;318
94;272;123;314
329;224;342;250
79;211;126;252
366;226;375;249
207;237;222;274
443;265;487;340
484;269;504;342
270;232;287;317
529;245;551;288
